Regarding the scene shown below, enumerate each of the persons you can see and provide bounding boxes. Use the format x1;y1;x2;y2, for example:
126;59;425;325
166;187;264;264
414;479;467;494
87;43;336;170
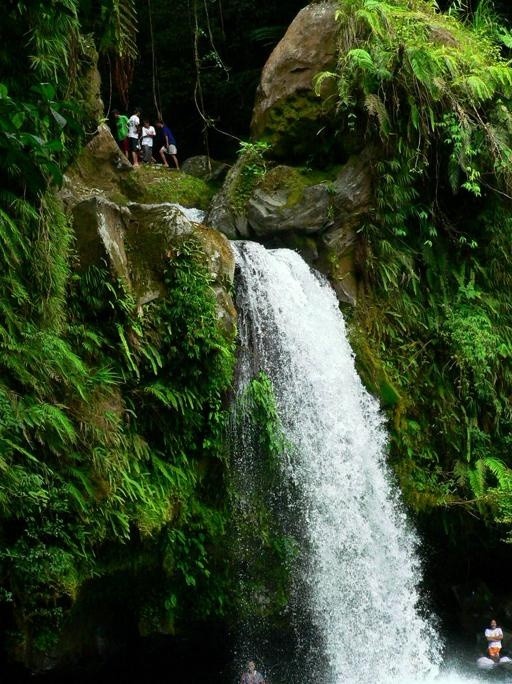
240;662;265;684
485;618;506;661
111;108;179;169
